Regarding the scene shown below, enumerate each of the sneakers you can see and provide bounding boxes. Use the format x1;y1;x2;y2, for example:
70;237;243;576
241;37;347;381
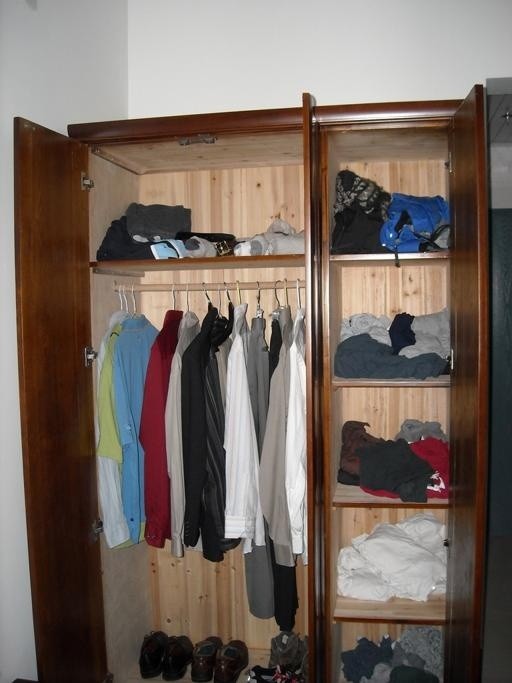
246;664;306;683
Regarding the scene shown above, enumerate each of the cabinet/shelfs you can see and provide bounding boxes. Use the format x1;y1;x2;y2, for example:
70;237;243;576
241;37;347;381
315;85;492;683
12;91;315;683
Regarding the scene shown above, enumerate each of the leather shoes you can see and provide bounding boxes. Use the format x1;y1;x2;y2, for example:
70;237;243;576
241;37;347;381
138;630;249;683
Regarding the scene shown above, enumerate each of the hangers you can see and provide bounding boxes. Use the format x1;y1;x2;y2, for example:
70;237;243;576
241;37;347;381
116;278;302;323
